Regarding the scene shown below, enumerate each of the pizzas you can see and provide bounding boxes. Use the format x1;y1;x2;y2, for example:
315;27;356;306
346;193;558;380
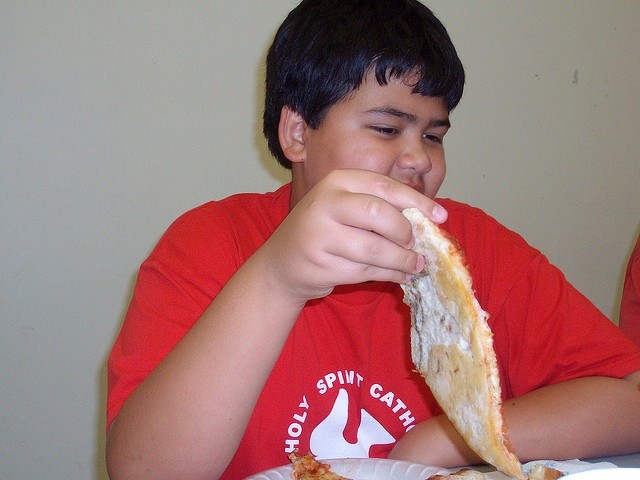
400;206;527;478
287;452;352;480
428;464;563;480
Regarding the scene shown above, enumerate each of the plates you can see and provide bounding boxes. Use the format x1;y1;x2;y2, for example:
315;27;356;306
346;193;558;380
245;457;451;480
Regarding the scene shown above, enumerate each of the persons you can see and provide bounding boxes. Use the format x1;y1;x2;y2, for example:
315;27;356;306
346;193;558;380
105;1;640;480
618;235;639;348
